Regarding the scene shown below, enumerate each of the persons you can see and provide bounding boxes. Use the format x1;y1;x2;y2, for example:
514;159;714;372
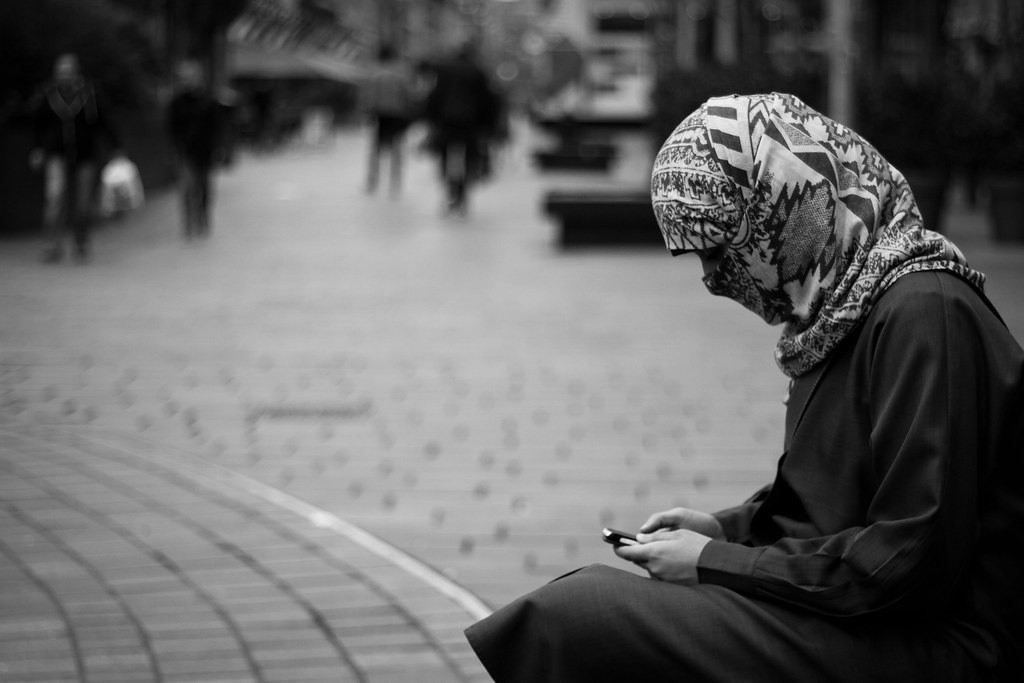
463;92;1023;683
365;35;509;211
30;50;236;267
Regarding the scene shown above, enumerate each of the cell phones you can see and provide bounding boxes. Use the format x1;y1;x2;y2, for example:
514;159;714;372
601;528;639;546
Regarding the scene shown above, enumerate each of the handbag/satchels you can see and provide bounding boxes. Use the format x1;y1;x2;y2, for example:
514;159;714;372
99;156;144;214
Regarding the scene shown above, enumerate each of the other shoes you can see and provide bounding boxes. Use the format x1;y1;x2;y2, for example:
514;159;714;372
37;244;63;263
72;245;88;264
